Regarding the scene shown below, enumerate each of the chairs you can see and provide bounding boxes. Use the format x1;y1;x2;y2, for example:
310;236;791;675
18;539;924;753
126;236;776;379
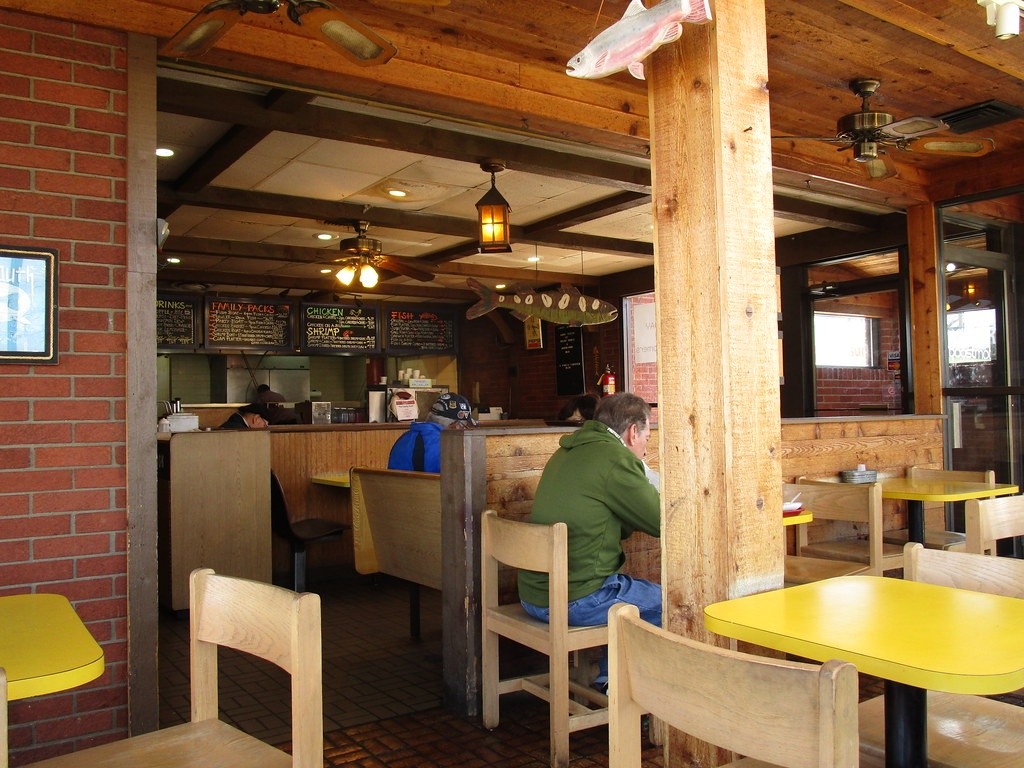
965;492;1024;557
859;541;1024;768
784;480;883;589
869;466;998;556
795;475;907;580
606;600;887;768
271;469;351;593
18;568;324;768
479;509;664;768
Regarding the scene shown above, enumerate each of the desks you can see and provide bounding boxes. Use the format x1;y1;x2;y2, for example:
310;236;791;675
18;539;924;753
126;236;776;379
312;472;351;488
704;574;1024;768
0;593;105;768
784;510;812;527
881;477;1019;550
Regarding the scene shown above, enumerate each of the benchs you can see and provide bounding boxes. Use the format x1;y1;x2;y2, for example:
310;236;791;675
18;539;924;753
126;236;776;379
349;466;443;642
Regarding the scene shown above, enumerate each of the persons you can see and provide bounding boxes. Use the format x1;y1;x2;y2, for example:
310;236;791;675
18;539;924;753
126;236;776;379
256;384;302;424
388;392;476;472
558;394;601;421
517;393;663;694
218;401;275;431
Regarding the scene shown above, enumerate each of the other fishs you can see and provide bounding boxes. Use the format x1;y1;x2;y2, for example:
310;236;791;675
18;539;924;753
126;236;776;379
465;275;618;327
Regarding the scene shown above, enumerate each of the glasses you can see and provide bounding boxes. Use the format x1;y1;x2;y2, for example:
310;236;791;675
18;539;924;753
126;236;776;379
450;421;473;431
260;416;269;429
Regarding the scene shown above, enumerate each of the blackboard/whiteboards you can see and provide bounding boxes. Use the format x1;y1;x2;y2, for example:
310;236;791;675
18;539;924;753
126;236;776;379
303;301;379;354
386;305;458;352
151;292;199;351
633;303;656;364
206;297;297;352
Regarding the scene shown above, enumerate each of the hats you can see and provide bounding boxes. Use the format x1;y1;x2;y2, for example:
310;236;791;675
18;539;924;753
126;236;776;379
237;401;272;423
430;392;478;427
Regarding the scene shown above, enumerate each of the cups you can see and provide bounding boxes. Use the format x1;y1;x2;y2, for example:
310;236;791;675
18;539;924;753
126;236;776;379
398;367;425;385
381;377;387;383
858;464;866;471
332;464;344;476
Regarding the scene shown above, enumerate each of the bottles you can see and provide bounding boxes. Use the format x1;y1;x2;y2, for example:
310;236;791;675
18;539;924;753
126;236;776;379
159;418;169;432
332;407;363;423
206;428;211;431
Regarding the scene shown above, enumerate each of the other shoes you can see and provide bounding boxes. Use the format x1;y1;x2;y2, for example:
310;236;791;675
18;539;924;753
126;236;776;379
589;680;648;732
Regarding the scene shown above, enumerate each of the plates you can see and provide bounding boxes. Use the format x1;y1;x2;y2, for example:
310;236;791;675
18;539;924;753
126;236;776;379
379;382;386;385
783;502;803;510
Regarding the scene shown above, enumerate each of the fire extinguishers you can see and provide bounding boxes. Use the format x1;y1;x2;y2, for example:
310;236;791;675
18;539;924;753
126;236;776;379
603;364;616;396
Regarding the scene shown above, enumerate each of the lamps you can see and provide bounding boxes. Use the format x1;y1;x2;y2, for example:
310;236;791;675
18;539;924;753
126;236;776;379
474;160;513;254
335;258;378;289
961;279;975;302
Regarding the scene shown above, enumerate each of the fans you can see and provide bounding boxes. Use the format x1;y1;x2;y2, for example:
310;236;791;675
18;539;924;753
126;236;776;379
305;221;437;288
771;80;995;182
159;0;398;67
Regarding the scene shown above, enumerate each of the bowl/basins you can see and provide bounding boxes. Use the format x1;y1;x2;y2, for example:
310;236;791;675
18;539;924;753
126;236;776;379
783;507;804;516
